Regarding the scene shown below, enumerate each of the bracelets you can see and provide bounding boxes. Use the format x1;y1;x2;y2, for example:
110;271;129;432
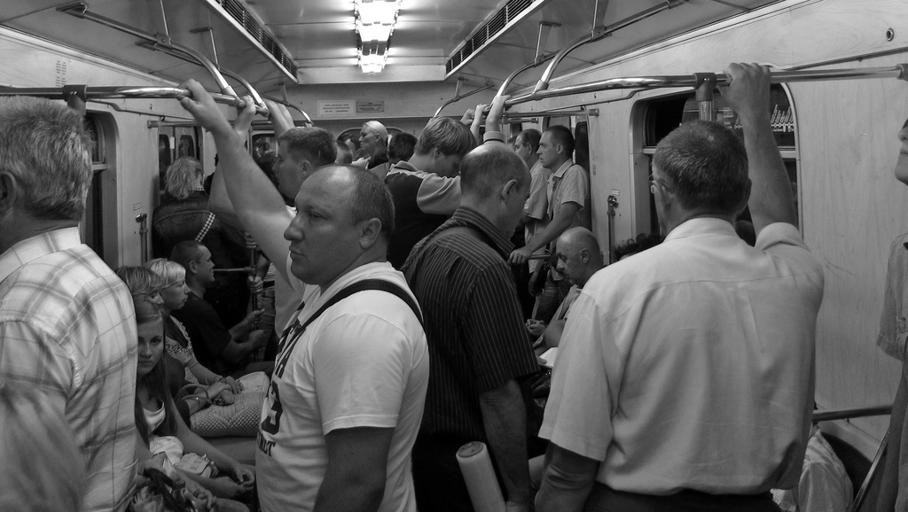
190;396;202;410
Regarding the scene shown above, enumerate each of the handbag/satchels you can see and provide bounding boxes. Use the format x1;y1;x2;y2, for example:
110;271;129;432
179;383;264;438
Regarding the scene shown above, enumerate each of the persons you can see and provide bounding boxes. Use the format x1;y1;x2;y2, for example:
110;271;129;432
277;102;295;129
385;133;417;173
337;133;361;161
470;104;487;144
533;226;603;366
1;96;140;512
113;266;255;464
170;239;267;377
207;92;337;234
133;300;256;499
397;140;534;512
507;125;589;265
533;62;823;512
460;109;475;126
177;78;430;512
142;258;245;395
261;98;289;140
350;120;388;170
873;106;907;512
153;156;252;330
514;129;551;274
384;94;512;270
129;445;249;511
